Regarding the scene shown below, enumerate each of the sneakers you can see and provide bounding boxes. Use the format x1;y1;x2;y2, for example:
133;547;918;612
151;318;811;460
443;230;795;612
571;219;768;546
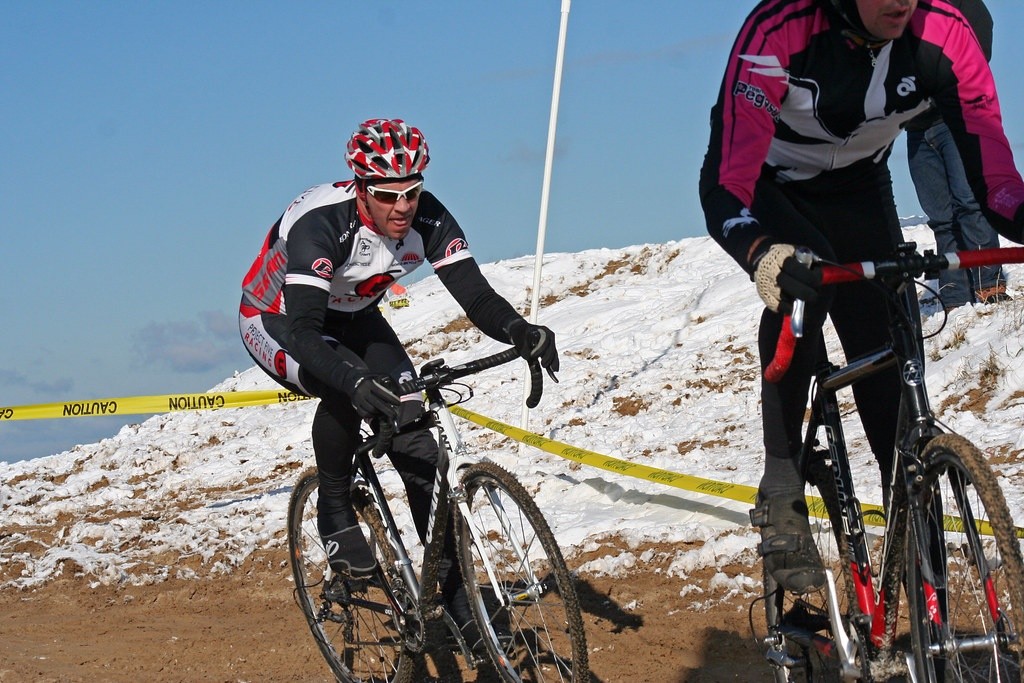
750;479;827;594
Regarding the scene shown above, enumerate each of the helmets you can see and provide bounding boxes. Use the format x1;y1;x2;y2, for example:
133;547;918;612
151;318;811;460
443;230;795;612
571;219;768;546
344;118;431;180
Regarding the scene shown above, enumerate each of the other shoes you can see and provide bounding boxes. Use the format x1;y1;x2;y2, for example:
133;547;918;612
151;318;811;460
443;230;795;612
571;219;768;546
316;505;376;574
982;293;1014;305
447;611;512;651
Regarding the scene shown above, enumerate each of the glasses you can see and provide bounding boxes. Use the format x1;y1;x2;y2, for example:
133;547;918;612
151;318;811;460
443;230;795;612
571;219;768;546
366;180;424;204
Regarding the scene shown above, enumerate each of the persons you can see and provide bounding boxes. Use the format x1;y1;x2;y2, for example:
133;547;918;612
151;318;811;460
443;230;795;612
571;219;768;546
698;0;1024;683
239;119;559;652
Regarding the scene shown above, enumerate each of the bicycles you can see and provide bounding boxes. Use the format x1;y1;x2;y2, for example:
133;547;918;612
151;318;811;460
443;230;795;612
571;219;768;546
748;237;1024;683
286;331;591;683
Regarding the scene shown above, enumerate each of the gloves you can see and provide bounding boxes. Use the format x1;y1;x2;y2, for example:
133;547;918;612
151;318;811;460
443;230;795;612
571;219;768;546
501;313;560;372
345;369;400;426
752;243;815;315
